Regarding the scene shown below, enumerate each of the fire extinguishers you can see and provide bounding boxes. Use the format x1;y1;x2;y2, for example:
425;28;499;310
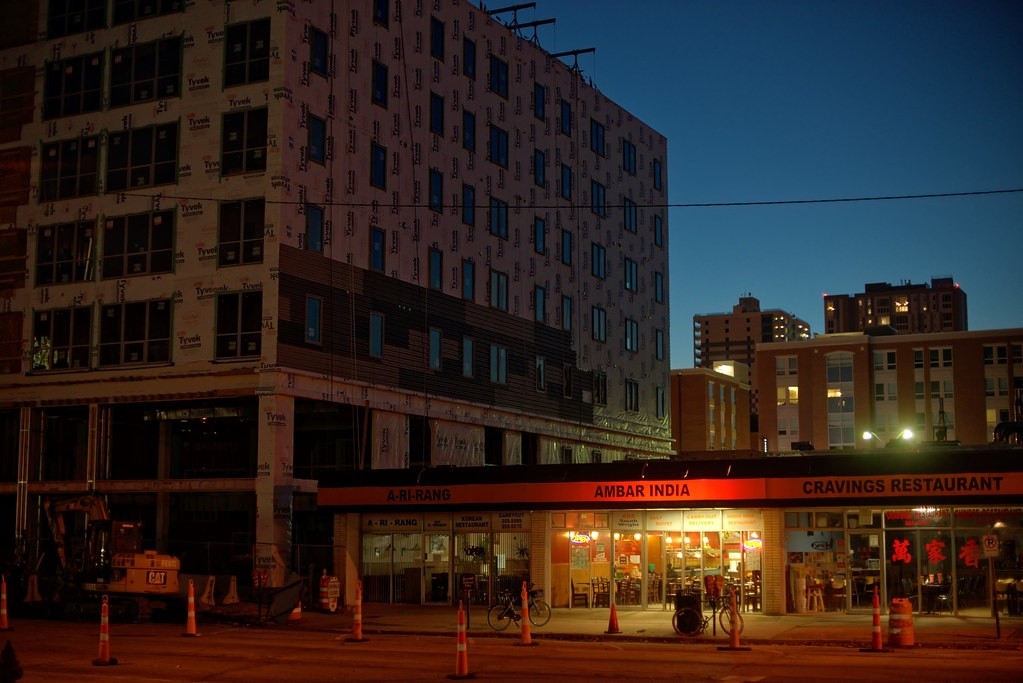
259;570;268;588
252;569;259;588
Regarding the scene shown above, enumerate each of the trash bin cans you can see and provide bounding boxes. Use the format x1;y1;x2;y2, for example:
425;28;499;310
672;589;703;633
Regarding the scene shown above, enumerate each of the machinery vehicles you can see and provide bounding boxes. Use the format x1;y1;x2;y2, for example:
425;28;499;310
40;492;181;624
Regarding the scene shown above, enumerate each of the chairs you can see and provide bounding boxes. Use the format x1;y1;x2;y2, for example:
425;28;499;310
805;574;880;613
935;585;953;613
570;576;762;614
901;578;924;610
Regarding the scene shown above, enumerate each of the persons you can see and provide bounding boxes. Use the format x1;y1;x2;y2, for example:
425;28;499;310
667;564;678;577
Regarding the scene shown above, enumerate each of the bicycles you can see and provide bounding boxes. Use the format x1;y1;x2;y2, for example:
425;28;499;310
672;589;743;636
487;583;551;631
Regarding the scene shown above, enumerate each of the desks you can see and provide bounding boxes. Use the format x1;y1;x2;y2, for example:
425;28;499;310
577;582;606;608
920;585;944;614
724;583;755;613
670;583;692;597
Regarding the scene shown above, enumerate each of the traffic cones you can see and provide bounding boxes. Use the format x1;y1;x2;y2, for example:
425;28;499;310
603;602;623;633
